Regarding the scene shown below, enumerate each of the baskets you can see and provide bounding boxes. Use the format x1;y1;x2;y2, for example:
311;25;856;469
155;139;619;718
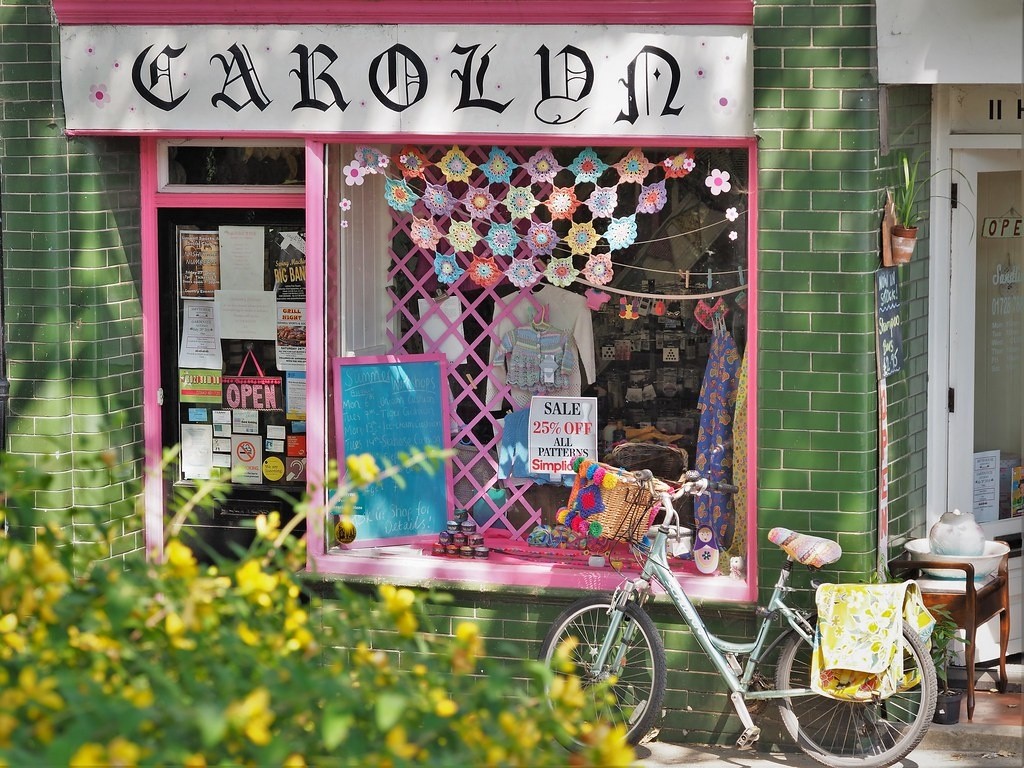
566;456;671;543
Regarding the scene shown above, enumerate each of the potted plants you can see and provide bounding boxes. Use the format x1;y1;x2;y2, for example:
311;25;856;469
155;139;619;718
890;151;976;264
926;605;970;725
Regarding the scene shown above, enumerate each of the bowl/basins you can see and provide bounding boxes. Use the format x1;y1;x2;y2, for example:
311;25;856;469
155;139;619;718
903;538;1011;578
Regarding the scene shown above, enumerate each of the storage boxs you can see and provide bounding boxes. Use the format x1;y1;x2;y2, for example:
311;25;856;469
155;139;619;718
566;456;672;544
646;525;694;557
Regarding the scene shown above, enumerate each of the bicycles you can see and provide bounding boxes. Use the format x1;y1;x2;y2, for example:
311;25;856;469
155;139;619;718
536;457;940;768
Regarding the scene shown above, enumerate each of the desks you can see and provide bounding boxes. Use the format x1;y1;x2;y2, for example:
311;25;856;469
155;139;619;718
889;543;1010;720
